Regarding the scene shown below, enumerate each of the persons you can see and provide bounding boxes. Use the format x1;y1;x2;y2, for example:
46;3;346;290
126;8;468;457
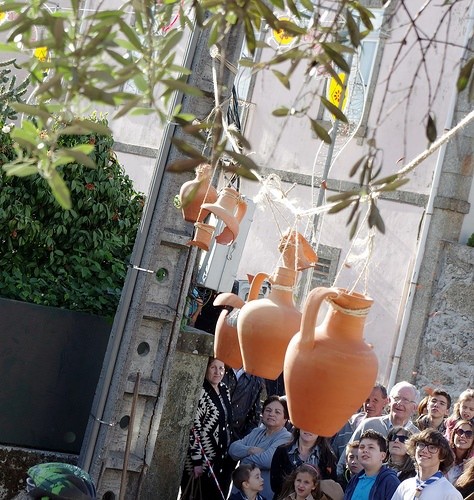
414;389;451;434
391;428;463;500
186;287;203;328
228;396;292;500
382;427;418;482
221;366;263;500
450;421;474;465
180;356;232;500
337;381;420;476
349;383;388;433
194;280;239;336
338;441;364;493
278;463;320;500
445;389;474;458
227;464;267;500
270;426;345;500
343;428;401;500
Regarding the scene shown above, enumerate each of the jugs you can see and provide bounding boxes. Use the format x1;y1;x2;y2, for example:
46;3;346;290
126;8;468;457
283;286;379;437
180;165;219;222
237;266;302;380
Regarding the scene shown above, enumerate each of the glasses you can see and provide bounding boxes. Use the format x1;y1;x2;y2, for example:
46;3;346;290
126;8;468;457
391;396;417;404
388;434;408;443
416;442;438;454
455;429;472;439
